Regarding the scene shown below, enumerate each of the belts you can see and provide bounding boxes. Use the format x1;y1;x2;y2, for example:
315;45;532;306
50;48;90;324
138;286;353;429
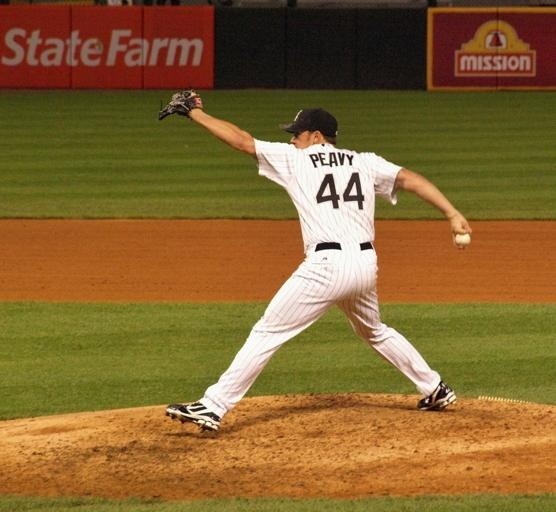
315;242;373;251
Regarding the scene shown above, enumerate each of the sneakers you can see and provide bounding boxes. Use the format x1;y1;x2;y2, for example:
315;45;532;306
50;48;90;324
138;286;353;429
167;403;221;428
417;383;457;411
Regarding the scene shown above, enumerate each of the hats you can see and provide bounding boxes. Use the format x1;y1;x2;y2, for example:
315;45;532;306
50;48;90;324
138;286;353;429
283;108;337;133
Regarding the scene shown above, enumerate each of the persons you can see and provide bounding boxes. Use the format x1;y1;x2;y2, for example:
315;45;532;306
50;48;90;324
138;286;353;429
158;88;472;432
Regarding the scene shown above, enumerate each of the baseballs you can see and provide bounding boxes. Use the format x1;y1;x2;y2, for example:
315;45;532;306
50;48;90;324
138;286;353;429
455;233;470;246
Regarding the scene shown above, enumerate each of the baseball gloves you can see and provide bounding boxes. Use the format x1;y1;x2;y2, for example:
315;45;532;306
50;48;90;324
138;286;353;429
158;89;203;120
